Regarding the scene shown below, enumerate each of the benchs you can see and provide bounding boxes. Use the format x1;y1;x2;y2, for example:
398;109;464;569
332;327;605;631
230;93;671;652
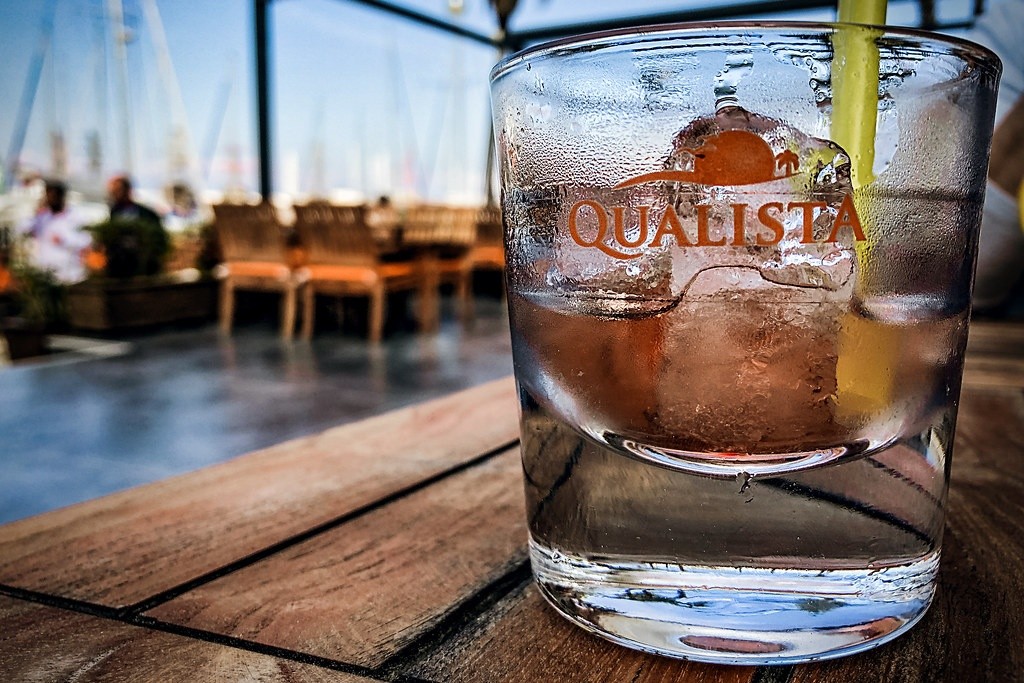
56;226;210;334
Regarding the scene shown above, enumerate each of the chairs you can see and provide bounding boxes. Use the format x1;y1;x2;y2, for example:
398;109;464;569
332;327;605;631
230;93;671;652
213;199;506;348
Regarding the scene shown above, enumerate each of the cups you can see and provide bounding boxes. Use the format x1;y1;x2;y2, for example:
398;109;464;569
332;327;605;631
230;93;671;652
488;18;1005;671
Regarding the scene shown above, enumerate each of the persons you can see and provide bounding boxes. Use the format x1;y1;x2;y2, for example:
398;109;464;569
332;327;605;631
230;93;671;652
844;1;1022;320
17;177;202;341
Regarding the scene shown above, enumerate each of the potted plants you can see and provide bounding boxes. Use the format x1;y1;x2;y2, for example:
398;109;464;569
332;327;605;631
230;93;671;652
0;226;64;360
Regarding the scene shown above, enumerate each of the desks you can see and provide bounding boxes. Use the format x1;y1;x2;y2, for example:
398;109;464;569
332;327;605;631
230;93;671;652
0;306;1024;683
